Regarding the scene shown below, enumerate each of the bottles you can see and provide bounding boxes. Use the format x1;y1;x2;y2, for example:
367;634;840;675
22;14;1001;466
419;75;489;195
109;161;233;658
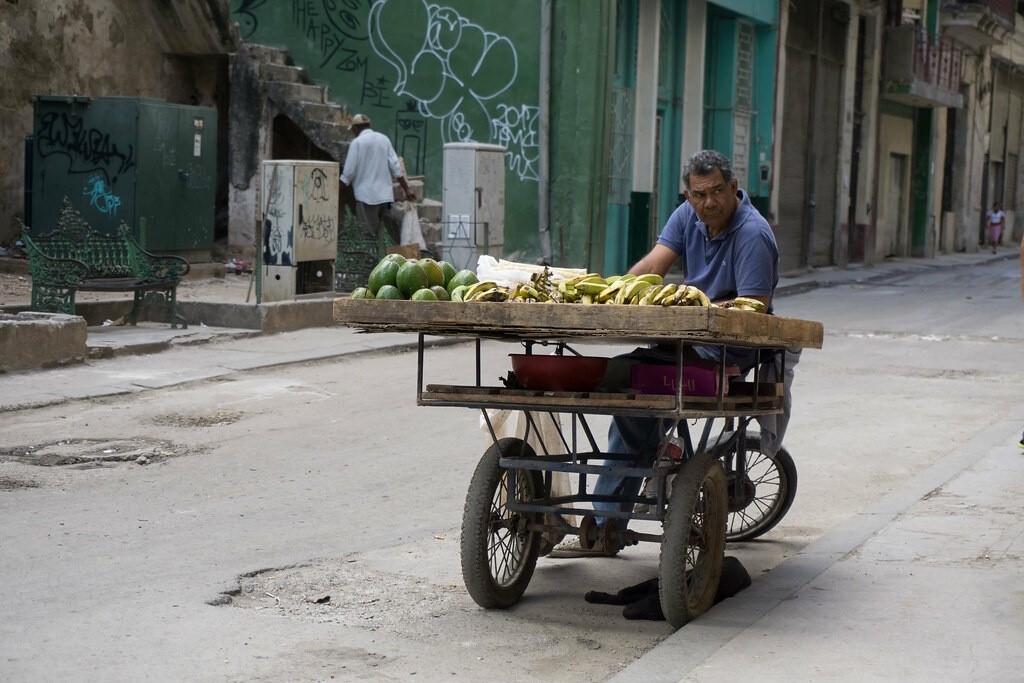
643;437;684;498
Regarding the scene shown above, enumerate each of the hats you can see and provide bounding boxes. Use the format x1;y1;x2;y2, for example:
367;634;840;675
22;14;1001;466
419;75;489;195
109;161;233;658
348;113;370;130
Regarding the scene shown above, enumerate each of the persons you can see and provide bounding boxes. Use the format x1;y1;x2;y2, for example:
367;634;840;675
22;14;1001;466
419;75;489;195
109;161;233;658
546;150;781;560
986;202;1005;254
339;114;418;252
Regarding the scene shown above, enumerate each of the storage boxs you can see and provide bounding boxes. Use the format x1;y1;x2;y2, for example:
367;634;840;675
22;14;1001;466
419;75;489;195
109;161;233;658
332;297;824;349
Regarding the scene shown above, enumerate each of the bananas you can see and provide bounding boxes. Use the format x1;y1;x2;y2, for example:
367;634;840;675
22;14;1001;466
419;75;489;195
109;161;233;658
451;272;764;312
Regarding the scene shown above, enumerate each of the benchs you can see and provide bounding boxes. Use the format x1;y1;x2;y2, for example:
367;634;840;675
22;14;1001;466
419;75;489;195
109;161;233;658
12;192;190;329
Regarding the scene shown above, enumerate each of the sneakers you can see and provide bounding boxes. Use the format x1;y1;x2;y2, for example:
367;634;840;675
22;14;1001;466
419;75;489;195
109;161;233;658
633;477;649;515
550;534;617;558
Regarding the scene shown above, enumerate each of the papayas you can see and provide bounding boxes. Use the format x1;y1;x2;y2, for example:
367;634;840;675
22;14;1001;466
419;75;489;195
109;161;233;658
349;254;479;300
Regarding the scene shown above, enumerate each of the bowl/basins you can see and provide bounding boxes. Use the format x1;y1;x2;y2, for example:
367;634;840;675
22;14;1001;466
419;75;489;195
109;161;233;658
509;354;610;390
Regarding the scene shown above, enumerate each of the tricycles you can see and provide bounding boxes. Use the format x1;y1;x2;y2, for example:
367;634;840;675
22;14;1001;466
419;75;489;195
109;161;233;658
333;295;826;630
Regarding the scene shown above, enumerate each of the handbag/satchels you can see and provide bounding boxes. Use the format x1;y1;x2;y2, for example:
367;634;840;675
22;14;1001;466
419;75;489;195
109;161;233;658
401;199;428;252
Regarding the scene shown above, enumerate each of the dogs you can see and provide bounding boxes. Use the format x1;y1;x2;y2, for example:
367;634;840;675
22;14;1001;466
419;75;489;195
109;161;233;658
585;556;752;622
